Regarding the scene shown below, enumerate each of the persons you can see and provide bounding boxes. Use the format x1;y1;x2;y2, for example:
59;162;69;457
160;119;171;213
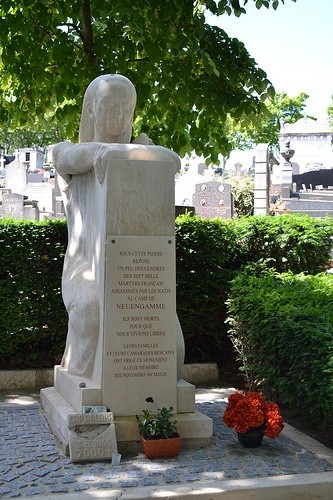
45;73;188;384
43;169;51;183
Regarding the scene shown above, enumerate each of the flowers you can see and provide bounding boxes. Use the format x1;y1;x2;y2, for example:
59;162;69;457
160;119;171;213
223;390;286;439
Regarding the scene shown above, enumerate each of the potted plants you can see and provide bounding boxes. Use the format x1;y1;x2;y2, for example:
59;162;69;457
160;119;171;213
135;403;182;460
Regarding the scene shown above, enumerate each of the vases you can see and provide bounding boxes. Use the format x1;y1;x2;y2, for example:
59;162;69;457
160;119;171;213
236;420;267;449
